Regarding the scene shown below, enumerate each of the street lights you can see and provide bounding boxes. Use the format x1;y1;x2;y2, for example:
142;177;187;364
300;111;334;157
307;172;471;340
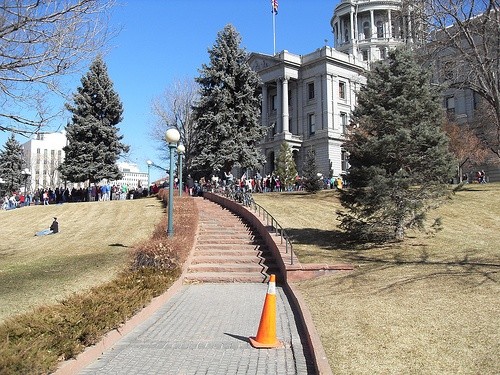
177;144;185;197
20;168;32;202
147;160;152;198
165;128;180;236
0;177;6;201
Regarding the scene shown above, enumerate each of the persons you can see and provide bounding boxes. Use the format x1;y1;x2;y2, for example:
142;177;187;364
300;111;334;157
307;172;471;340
463;173;470;183
32;216;62;238
172;171;310;203
323;176;343;189
0;184;171;212
475;169;488;183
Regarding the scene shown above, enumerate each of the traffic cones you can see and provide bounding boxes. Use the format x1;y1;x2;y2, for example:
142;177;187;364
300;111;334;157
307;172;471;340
249;273;283;349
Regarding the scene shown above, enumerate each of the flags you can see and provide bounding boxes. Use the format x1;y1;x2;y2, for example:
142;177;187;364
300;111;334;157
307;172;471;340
270;0;279;16
